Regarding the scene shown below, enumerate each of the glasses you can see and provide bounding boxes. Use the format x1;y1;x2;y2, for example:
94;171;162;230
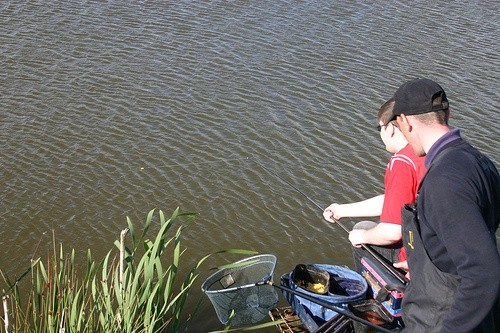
377;126;388;130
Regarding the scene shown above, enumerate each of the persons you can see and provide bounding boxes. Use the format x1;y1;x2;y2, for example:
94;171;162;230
323;98;428;274
382;78;500;333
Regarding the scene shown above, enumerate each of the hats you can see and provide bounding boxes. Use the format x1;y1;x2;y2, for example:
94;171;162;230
384;77;449;126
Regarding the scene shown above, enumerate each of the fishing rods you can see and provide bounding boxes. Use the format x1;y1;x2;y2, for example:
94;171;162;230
246;157;408;285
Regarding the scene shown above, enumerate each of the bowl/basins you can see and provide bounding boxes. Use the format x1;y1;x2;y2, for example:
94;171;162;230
348;300;394;333
293;264;330;295
328;276;365;296
